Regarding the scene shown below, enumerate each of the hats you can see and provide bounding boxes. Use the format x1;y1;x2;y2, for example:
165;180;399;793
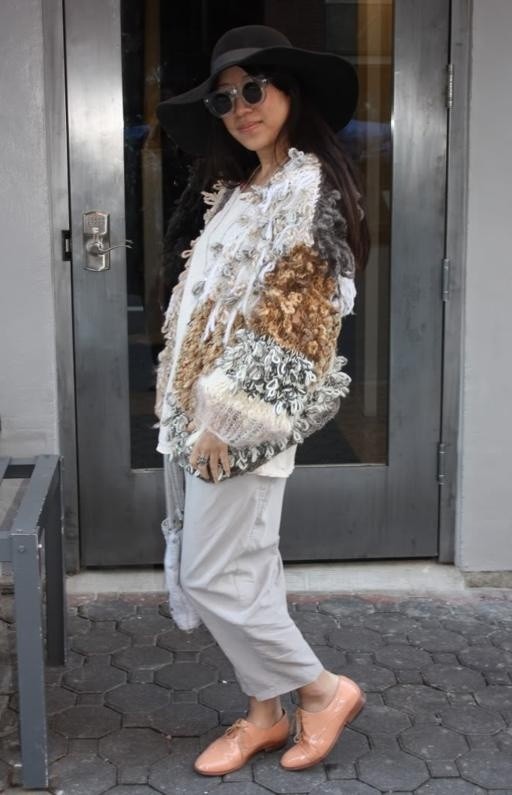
154;24;361;159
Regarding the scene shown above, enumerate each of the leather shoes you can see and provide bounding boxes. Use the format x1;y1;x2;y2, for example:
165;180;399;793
280;673;367;771
194;704;291;778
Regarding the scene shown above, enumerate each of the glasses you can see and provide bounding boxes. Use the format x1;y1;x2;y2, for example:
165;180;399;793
202;73;274;120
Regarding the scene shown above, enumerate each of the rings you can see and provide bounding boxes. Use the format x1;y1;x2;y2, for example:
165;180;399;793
196;457;206;464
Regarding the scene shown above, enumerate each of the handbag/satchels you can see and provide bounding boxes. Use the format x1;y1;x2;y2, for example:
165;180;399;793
158;502;202;633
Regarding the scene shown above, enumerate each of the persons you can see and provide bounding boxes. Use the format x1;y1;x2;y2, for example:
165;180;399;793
150;26;373;781
